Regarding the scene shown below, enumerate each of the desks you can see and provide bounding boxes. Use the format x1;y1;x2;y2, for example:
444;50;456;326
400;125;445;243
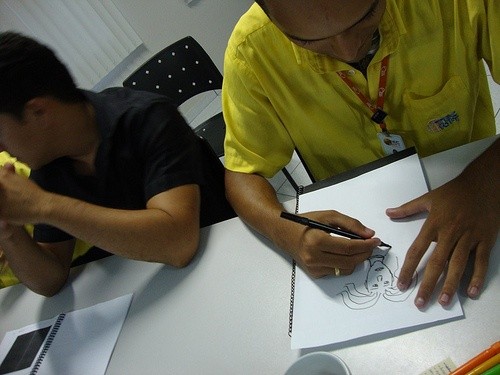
0;138;499;375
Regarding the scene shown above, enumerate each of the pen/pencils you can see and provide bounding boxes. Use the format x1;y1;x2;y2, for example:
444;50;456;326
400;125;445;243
280;211;393;250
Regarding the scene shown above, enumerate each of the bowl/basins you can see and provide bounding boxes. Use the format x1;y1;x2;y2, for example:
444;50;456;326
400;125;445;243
283;352;352;375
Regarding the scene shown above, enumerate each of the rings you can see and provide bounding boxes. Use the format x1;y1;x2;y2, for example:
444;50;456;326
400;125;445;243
335;268;339;276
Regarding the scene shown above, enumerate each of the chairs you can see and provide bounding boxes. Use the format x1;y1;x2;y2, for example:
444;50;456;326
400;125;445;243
122;36;300;211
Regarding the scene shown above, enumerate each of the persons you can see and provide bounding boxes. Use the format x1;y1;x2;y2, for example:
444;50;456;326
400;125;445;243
0;31;238;296
222;1;500;307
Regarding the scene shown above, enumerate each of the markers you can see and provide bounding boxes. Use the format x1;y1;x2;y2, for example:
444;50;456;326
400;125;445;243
449;340;500;375
